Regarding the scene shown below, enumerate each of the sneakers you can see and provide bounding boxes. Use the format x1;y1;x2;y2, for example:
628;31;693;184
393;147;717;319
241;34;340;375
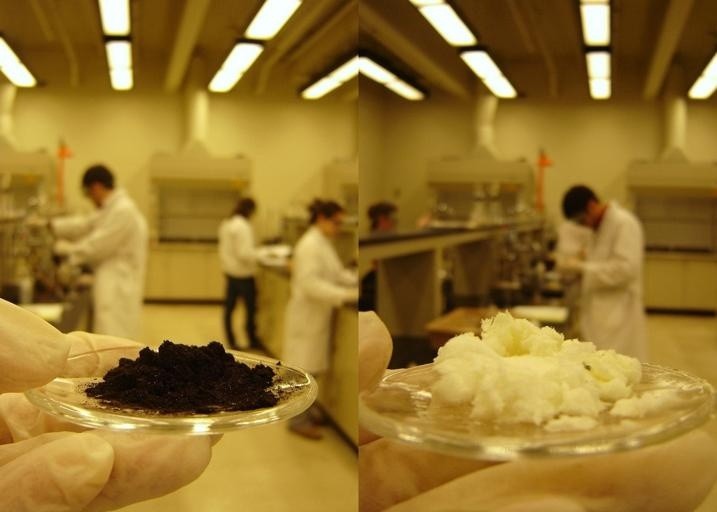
290;421;322;440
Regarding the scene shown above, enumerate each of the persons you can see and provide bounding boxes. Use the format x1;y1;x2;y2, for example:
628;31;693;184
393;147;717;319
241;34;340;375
363;202;399;320
48;163;151;340
216;199;279;352
555;185;647;358
285;198;360;439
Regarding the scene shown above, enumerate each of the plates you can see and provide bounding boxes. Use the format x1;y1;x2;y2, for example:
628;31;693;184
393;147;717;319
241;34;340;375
26;341;317;436
360;355;715;465
